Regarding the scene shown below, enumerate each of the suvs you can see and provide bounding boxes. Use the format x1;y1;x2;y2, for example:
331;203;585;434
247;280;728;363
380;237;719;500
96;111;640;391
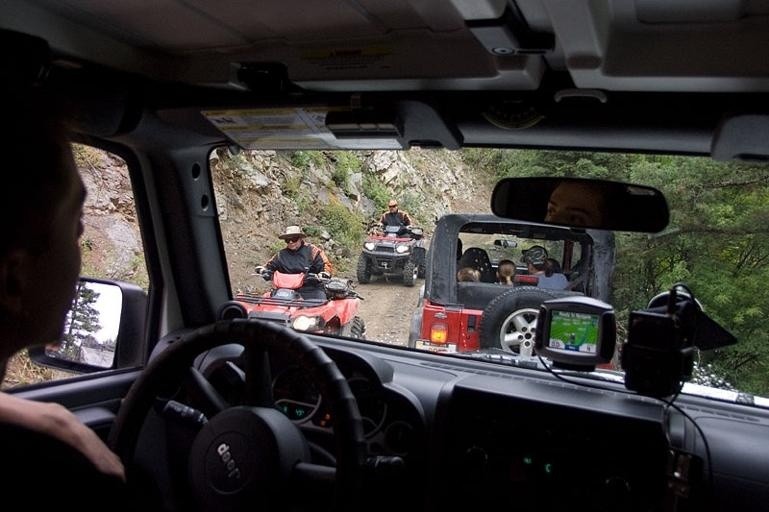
406;212;616;369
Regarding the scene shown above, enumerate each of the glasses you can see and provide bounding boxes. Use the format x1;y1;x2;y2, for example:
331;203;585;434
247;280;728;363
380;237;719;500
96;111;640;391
389;205;398;208
284;236;300;243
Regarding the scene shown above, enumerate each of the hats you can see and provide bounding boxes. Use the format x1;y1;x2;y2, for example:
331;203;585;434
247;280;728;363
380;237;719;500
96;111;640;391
519;244;549;265
278;225;306;239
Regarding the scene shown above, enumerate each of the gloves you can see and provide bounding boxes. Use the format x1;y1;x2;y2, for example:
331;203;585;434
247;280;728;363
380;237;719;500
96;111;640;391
255;265;267;276
318;270;331;284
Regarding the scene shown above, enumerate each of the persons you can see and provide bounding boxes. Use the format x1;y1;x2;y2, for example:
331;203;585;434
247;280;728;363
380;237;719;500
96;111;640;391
457;266;481;283
255;226;332;302
375;200;415;239
548;259;564;271
543;178;632;229
492;261;517;288
521;247;571;293
0;31;131;512
